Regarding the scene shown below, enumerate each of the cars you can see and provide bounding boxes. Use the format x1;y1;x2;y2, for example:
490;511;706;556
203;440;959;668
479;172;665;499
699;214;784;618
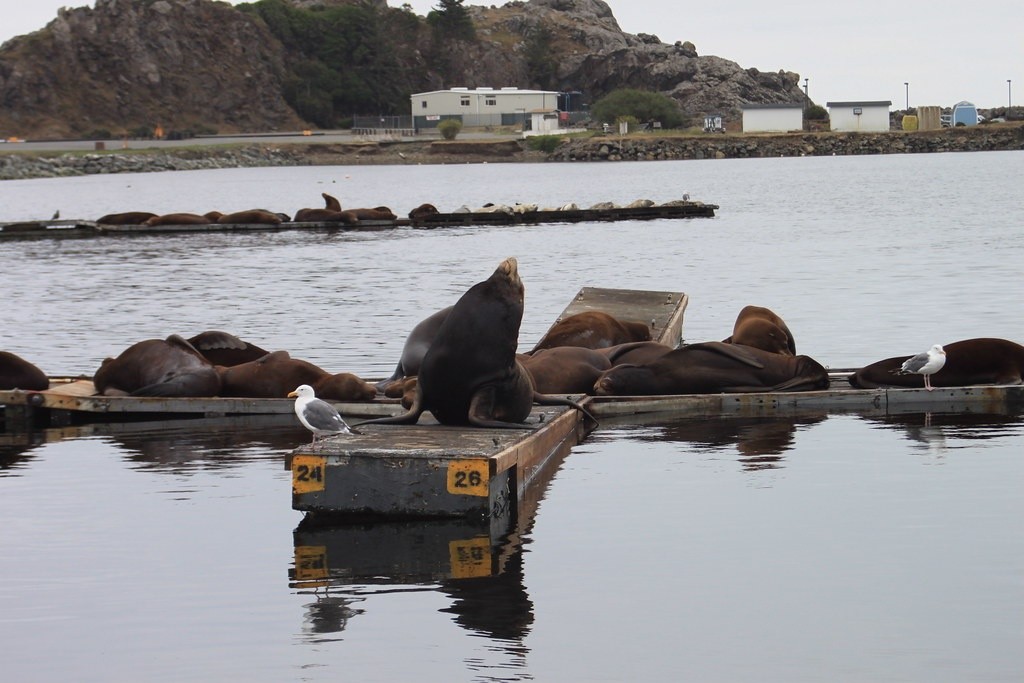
941;114;986;128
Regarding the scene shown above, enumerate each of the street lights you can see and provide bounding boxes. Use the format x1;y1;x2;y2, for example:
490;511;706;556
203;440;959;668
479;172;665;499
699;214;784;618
904;83;909;113
802;78;807;118
1007;79;1012;120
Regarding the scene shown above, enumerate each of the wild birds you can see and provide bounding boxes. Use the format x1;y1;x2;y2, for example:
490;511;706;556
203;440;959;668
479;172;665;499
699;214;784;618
682;191;690;202
287;384;366;449
482;202;495;212
52;209;59;219
887;343;947;391
515;202;522;206
